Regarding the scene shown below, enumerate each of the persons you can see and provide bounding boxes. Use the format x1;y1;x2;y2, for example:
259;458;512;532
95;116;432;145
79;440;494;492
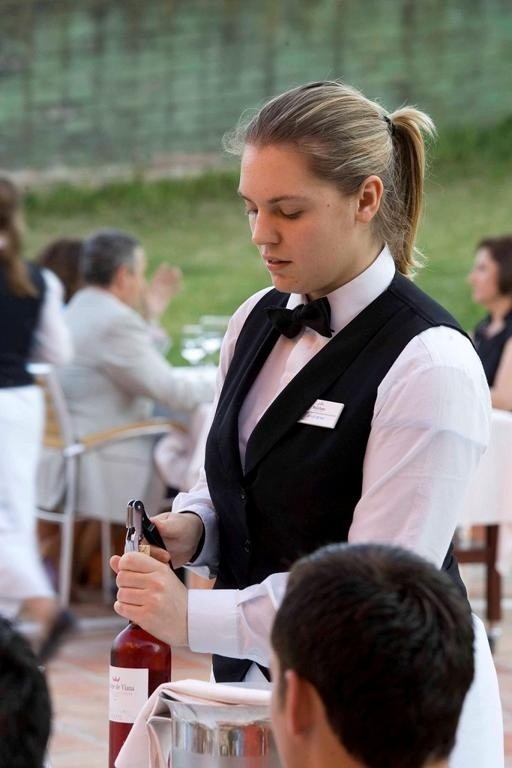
0;618;53;768
270;543;475;765
36;238;105;595
109;80;506;765
463;235;512;414
35;230;221;521
1;176;77;664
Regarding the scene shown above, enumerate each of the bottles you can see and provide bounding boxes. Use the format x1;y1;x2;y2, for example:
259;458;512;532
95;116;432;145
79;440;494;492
107;621;173;768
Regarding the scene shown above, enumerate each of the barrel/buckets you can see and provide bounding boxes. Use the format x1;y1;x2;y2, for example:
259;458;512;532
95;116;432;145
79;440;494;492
159;694;281;768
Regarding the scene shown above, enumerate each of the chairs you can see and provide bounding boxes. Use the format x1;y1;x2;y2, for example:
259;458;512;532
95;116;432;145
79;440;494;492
28;361;197;630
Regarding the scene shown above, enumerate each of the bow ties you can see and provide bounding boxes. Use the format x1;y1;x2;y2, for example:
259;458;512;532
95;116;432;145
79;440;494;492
266;296;333;342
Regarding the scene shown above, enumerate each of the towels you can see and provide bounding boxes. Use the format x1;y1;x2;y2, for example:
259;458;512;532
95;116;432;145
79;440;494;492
112;678;270;768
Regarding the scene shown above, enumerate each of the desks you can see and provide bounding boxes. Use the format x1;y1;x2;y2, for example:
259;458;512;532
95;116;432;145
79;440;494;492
453;408;511;621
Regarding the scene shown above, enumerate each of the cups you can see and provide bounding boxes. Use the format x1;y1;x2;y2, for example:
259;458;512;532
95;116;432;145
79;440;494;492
180;315;229;367
